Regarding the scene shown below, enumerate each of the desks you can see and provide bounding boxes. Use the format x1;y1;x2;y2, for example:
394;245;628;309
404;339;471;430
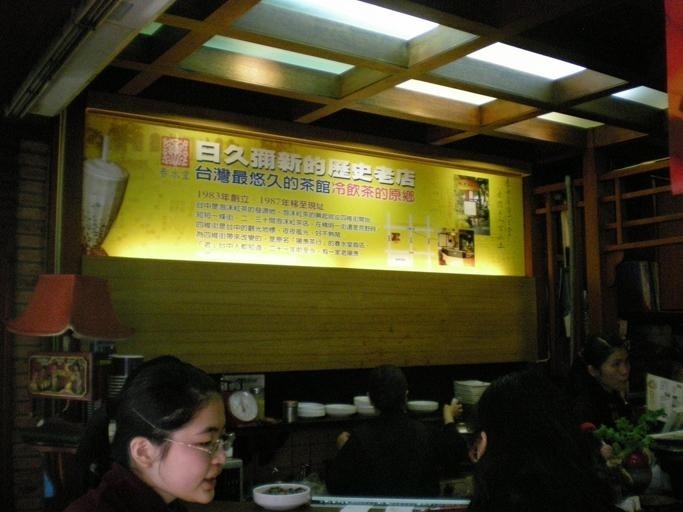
279;410;451;428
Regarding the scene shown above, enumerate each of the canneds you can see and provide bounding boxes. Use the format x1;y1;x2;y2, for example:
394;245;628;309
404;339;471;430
282;400;298;423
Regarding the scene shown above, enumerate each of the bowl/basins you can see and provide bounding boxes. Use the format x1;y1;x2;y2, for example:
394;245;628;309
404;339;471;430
441;247;474;267
650;438;683;475
250;483;311;510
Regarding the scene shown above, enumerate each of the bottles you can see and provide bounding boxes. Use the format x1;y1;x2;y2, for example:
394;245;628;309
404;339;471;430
252;387;267;420
282;399;296;422
624;452;651;494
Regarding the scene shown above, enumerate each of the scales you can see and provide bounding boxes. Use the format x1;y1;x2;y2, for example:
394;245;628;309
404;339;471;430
219;375;259;428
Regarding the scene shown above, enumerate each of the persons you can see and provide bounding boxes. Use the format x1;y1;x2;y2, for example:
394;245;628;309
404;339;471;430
456;362;620;512
574;337;633;428
64;353;226;511
322;353;467;501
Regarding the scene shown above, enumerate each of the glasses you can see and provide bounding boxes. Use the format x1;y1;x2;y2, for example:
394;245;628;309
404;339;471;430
162;431;236;457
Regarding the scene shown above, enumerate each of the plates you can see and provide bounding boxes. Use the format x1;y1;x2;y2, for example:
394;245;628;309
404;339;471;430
454;377;491;406
297;395;440;418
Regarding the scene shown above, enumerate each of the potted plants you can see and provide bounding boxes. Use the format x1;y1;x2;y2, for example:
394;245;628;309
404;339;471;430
589;403;670;497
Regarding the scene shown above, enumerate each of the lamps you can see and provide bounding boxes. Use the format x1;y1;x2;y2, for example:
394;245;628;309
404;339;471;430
7;269;137;444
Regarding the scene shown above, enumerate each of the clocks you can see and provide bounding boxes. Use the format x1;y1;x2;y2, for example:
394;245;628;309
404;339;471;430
227;389;259;423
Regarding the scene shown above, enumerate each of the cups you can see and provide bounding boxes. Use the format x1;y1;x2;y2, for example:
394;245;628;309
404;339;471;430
105;354;142;398
83;157;129;248
